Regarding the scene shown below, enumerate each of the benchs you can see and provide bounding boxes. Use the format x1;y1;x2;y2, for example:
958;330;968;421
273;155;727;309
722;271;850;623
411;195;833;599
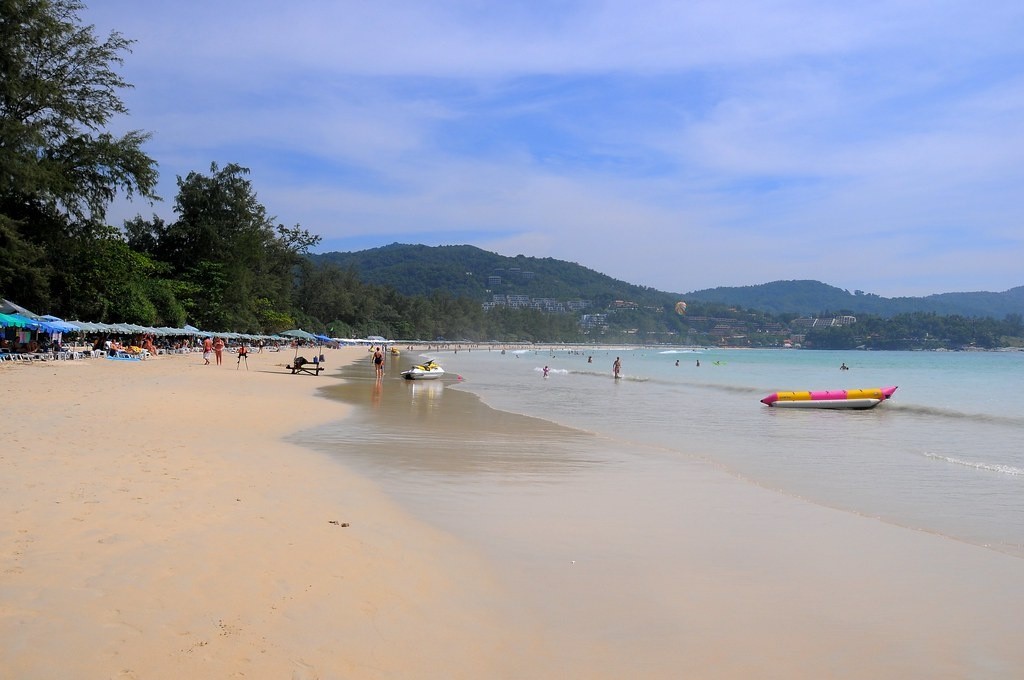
286;366;324;370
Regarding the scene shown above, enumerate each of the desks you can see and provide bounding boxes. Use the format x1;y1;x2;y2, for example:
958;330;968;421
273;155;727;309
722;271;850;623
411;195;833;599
291;362;319;376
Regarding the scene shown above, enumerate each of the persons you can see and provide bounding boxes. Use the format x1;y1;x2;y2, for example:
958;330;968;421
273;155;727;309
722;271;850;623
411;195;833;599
588;356;592;363
543;366;548;377
675;360;679;367
840;362;846;370
0;333;387;381
613;357;621;379
696;359;700;367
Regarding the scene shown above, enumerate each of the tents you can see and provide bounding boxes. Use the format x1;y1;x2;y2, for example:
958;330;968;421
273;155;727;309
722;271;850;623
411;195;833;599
0;299;332;343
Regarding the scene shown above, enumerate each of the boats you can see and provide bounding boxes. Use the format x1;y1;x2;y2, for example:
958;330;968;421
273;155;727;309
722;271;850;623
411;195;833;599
760;386;899;410
401;359;442;380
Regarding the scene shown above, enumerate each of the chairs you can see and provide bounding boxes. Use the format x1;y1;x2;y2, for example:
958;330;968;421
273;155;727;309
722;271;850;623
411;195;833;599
0;346;289;363
